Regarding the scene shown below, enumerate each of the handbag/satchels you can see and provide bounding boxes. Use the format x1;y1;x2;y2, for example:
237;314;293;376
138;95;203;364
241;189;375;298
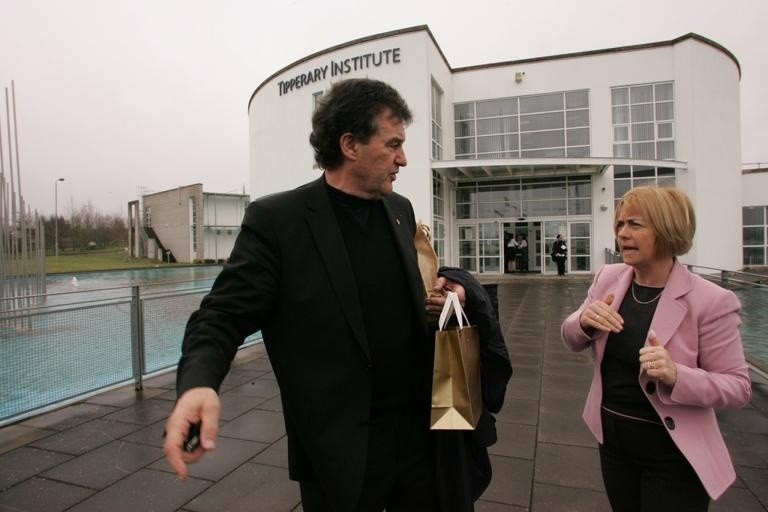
413;223;482;430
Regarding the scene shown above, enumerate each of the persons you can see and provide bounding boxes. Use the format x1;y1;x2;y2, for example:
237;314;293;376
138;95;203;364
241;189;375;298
506;233;519;272
518;233;528;271
559;185;754;511
553;233;569;277
162;77;491;511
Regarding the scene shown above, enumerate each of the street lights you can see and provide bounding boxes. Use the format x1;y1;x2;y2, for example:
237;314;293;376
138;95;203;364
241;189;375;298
55;178;65;257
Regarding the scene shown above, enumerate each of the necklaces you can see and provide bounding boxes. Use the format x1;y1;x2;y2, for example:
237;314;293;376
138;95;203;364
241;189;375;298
630;282;664;304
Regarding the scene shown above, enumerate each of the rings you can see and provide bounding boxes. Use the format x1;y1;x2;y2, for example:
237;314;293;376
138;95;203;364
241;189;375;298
594;314;600;321
648;360;654;368
